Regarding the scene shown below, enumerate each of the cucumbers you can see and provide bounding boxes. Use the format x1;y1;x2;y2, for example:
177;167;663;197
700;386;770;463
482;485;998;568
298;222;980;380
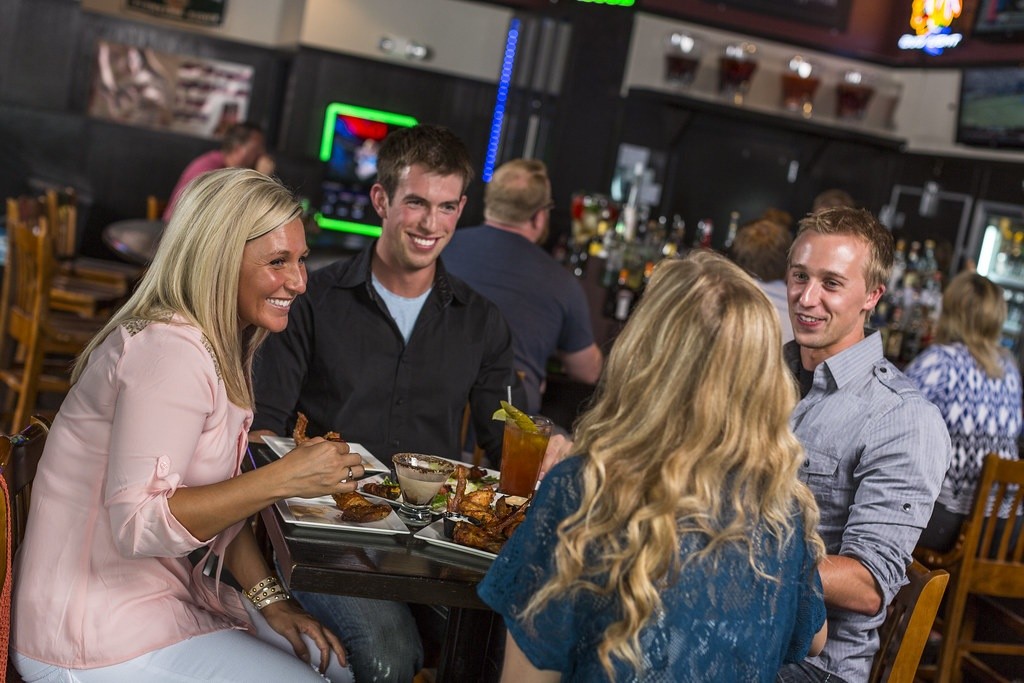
501;400;539;434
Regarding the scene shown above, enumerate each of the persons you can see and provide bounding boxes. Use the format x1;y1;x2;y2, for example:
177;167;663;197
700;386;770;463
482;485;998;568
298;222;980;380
438;159;603;458
477;251;828;683
162;120;277;223
903;270;1024;558
240;123;529;683
777;208;953;683
732;207;794;284
9;167;365;683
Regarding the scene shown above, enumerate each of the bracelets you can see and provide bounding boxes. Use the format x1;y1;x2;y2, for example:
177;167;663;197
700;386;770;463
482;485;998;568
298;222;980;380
242;575;289;609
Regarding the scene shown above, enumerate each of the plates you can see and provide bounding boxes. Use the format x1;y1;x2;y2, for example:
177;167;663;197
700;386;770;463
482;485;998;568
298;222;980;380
274;485;412;536
259;435;392;476
412;492;532;561
348;455;505;517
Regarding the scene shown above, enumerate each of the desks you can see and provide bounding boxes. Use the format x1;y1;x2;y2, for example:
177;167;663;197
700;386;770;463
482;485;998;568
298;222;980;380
241;440;530;682
104;217;172;284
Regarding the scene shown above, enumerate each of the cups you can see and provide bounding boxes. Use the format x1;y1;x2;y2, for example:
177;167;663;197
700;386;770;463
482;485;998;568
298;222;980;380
392;451;455;527
781;54;829;119
499;413;555;498
717;41;763;101
664;31;709;91
836;65;876;126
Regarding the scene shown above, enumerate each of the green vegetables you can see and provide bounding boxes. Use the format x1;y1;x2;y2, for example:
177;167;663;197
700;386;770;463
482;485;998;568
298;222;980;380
381;471;499;511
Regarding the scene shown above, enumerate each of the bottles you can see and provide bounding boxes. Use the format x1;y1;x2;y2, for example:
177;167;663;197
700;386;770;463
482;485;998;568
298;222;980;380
571;189;1019;369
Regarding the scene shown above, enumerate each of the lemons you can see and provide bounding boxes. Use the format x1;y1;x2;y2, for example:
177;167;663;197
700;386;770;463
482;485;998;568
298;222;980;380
492;409;509;421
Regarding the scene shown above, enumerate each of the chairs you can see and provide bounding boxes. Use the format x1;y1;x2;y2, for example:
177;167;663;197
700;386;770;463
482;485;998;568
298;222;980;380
0;185;131;682
870;559;950;683
932;452;1024;683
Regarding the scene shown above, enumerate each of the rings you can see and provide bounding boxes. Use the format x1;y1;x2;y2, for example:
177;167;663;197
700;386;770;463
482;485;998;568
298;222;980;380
349;467;353;480
